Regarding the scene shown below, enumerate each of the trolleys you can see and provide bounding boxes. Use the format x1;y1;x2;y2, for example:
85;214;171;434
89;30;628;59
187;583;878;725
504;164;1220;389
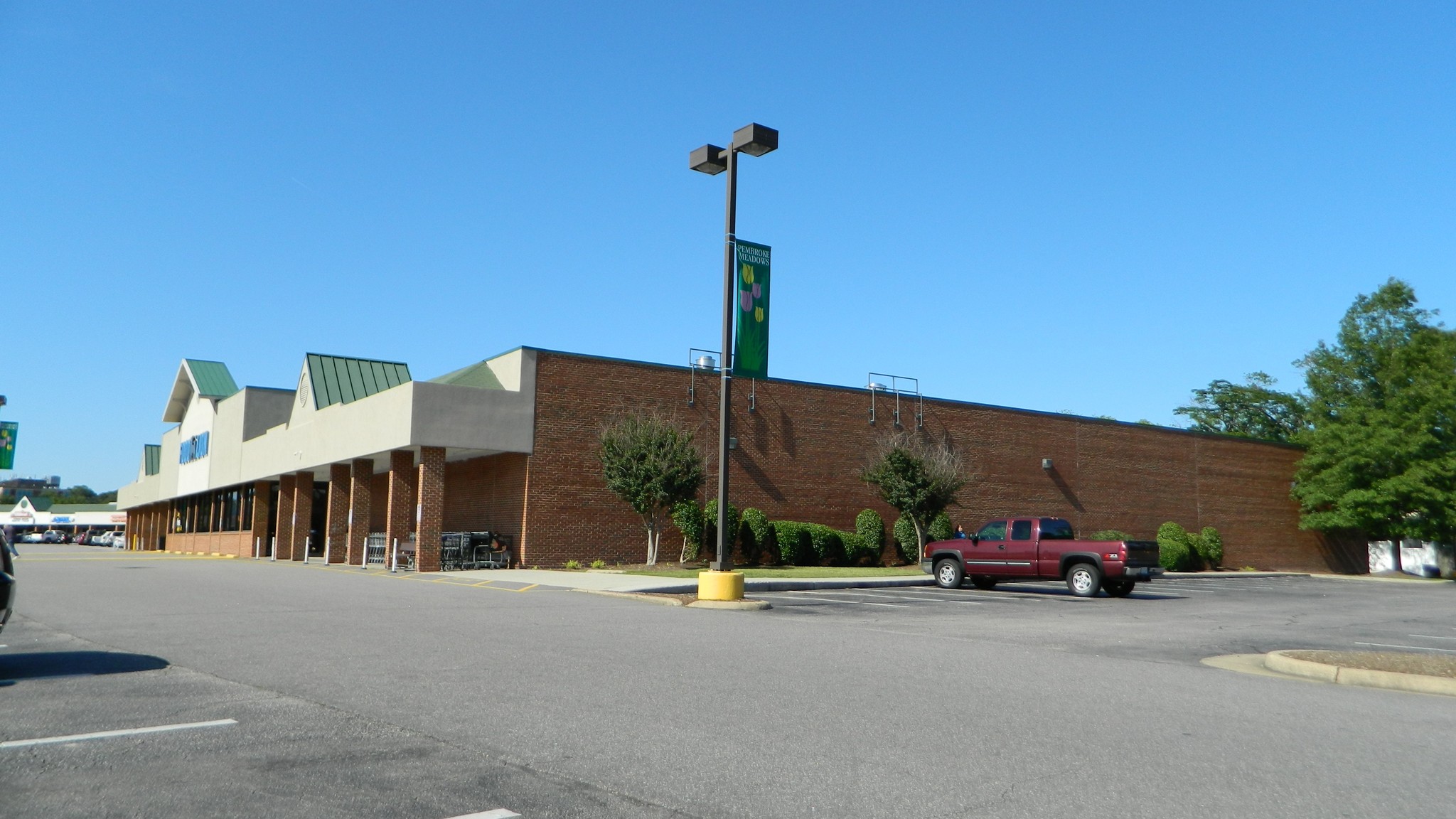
409;534;465;572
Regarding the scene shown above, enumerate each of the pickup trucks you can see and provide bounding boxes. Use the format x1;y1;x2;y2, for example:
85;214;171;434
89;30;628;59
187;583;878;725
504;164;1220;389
22;529;66;544
920;515;1165;598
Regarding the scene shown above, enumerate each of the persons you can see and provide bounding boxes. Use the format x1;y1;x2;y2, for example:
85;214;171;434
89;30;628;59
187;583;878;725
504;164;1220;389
3;524;20;560
954;524;965;539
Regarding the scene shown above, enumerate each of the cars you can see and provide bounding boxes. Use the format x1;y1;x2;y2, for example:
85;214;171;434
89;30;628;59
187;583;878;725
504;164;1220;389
63;529;126;548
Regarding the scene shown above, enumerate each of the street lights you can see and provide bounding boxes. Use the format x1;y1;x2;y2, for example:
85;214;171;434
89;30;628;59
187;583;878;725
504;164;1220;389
687;121;781;572
